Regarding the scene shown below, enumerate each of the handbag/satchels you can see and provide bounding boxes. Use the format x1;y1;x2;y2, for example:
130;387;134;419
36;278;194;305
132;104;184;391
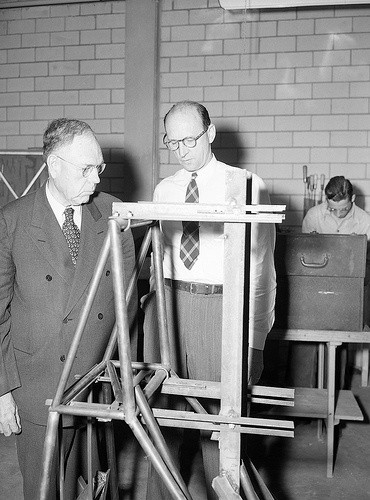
273;233;367;332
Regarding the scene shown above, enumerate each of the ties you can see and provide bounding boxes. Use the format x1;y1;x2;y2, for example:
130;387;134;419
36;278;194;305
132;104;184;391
180;173;199;269
61;208;80;269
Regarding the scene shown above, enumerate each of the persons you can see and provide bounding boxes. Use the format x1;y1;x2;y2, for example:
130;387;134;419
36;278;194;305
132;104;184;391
0;117;142;500
139;101;277;500
289;175;370;425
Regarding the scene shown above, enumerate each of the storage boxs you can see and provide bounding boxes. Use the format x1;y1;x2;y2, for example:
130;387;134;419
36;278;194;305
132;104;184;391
273;232;367;330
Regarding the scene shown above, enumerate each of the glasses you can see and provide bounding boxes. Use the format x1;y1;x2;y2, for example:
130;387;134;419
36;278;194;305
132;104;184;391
327;205;350;211
52;153;106;178
163;129;207;151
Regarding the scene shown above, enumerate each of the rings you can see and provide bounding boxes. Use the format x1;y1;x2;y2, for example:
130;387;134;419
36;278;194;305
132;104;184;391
255;376;261;381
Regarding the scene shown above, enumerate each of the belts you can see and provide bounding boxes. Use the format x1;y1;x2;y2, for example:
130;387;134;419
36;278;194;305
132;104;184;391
164;278;223;295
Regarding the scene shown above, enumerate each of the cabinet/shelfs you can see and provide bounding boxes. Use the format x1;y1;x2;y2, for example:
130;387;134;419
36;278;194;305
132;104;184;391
265;330;370;476
